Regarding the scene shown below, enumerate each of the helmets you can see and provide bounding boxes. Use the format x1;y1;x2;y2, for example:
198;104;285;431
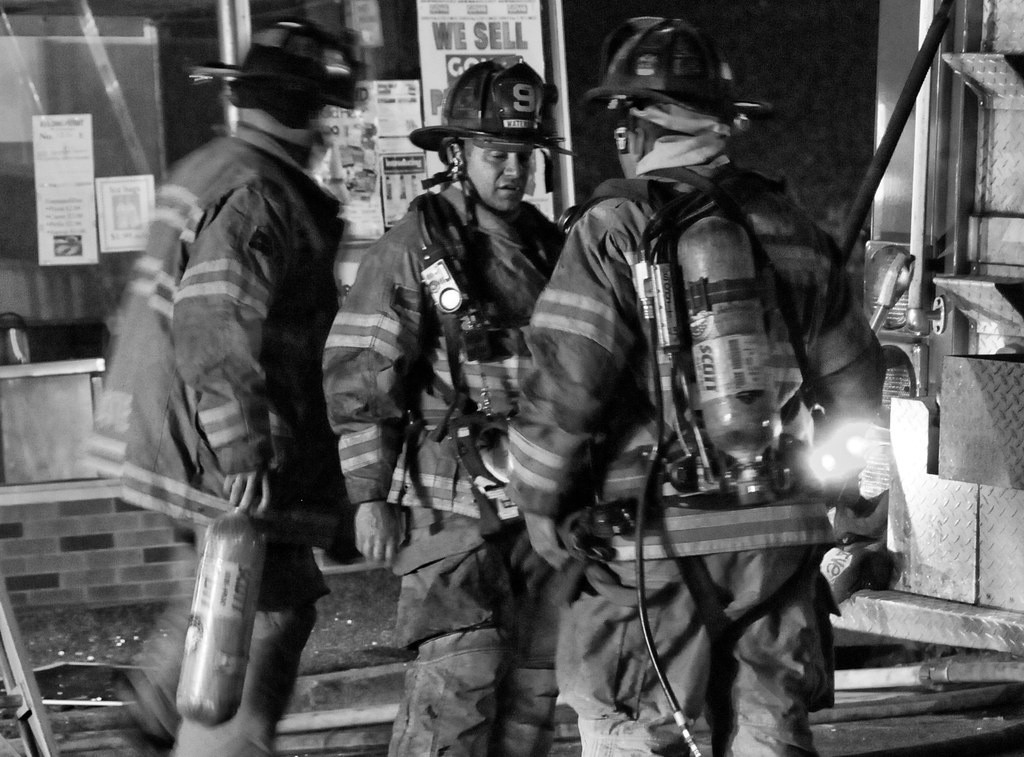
229;17;355;128
408;55;559;151
585;16;773;118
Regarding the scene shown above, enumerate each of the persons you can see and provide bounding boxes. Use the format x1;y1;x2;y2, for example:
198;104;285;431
502;15;887;757
322;58;565;756
77;17;369;757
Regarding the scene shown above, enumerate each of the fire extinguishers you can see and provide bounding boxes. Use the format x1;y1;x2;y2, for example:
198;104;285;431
174;487;270;728
673;217;784;506
